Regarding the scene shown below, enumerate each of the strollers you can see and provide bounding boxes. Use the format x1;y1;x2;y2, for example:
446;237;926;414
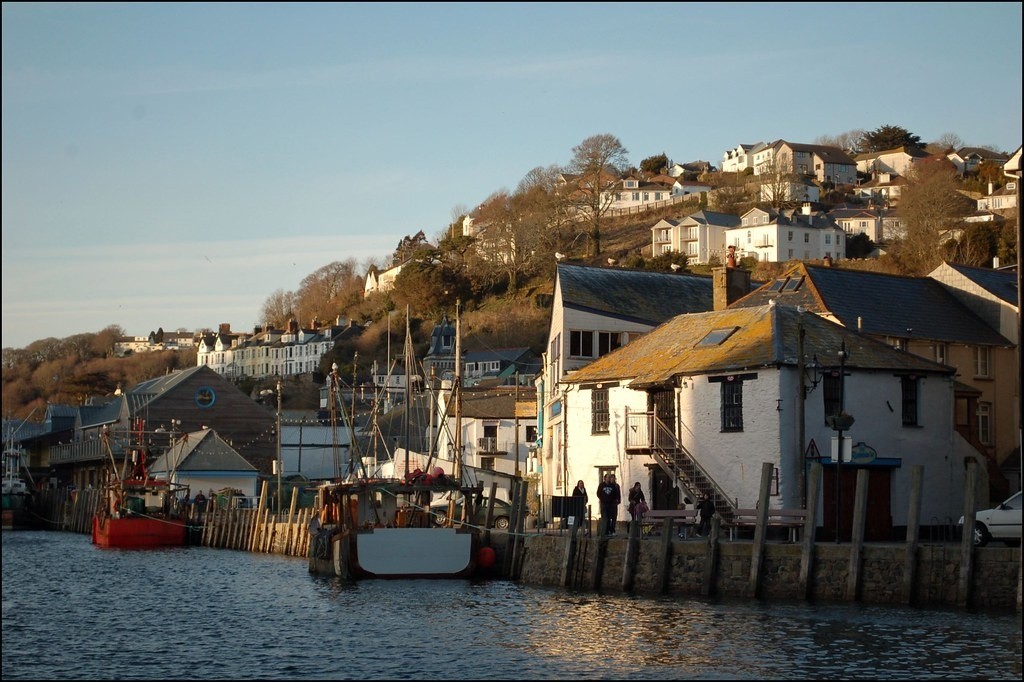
633;501;662;537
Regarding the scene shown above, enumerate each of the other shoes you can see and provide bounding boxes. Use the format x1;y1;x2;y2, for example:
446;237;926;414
696;534;702;537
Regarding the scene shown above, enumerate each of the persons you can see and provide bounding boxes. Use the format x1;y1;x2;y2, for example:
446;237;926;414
678;503;686;539
572;480;588;527
235;490;246;509
597;473;621;537
628;482;646;520
194;489;205;521
696;491;715;538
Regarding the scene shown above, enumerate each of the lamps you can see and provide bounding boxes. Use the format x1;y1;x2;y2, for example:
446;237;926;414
804;353;825;399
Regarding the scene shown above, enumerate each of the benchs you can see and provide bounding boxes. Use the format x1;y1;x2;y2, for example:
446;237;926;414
729;510;807;543
636;510;698;541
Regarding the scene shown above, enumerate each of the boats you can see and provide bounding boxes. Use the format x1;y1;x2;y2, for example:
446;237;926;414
3;407;40;532
93;417;189;548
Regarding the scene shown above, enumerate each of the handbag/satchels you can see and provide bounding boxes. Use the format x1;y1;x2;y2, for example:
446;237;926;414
627;504;630;510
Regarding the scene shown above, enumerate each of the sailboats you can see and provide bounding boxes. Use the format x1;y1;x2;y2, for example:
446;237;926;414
328;300;484;579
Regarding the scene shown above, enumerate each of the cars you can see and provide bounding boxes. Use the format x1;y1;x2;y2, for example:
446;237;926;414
429;493;510;530
954;491;1021;549
